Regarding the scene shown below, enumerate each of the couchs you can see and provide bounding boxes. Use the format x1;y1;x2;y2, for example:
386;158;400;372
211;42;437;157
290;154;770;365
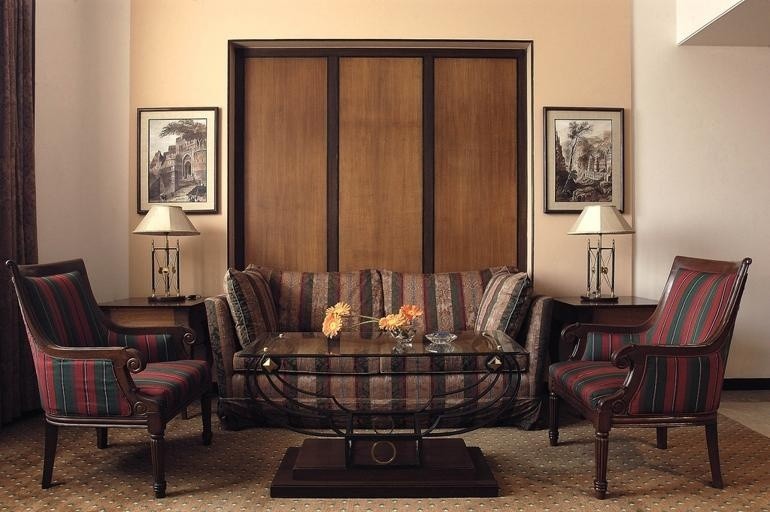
202;262;554;430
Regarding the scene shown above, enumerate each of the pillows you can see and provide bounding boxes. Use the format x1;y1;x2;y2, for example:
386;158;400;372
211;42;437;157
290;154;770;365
265;269;381;332
224;262;281;344
475;266;534;336
381;270;492;332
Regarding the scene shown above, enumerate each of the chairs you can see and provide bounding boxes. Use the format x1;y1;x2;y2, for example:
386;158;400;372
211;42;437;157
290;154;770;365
5;258;213;499
547;257;753;501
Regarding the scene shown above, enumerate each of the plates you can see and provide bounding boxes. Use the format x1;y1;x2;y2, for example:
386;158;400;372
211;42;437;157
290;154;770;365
424;333;458;344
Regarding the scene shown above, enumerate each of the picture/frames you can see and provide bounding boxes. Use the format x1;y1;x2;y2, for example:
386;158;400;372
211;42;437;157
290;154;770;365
135;106;221;216
542;107;626;215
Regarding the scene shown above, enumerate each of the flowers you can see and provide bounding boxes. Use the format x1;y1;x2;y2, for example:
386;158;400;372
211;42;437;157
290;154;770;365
321;301;424;338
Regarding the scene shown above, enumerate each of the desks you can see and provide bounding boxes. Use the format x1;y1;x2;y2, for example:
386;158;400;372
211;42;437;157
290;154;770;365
95;294;215;424
552;293;661;323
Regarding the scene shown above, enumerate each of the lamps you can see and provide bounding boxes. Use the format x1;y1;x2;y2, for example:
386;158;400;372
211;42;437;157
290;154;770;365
131;203;202;303
567;207;636;297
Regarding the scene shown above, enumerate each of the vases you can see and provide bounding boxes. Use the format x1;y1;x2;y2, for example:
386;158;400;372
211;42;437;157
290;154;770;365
390;327;415;351
